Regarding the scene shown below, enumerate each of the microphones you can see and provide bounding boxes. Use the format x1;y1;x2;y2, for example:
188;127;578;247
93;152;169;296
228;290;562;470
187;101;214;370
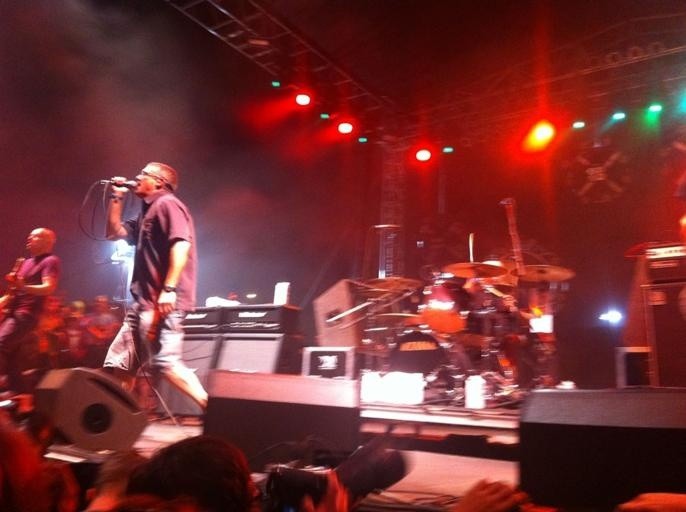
100;179;138;189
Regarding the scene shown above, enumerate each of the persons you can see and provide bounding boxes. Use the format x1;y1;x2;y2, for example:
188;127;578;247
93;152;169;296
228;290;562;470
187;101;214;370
619;492;685;512
0;228;62;389
87;439;349;512
33;295;121;351
452;477;528;512
1;409;79;512
102;162;209;414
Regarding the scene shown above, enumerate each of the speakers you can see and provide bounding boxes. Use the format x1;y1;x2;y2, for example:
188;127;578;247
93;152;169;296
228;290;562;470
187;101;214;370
519;389;686;508
154;335;220;417
34;367;148;452
207;369;359;473
214;334;306;375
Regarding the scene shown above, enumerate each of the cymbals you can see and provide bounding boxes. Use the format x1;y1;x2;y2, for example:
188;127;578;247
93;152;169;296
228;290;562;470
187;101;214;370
440;261;509;278
510;264;576;284
361;277;420;291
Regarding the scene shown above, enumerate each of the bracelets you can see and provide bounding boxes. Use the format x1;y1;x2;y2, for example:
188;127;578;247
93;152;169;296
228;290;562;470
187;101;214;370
110;194;124;202
162;286;177;292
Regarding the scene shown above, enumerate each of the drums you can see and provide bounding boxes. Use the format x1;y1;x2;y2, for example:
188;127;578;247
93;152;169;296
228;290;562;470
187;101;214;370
423;277;471;335
459;306;495;346
418;285;433;315
388;329;451;386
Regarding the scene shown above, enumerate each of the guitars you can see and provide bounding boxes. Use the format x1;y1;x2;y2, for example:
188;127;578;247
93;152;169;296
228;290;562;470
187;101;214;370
0;258;26;324
